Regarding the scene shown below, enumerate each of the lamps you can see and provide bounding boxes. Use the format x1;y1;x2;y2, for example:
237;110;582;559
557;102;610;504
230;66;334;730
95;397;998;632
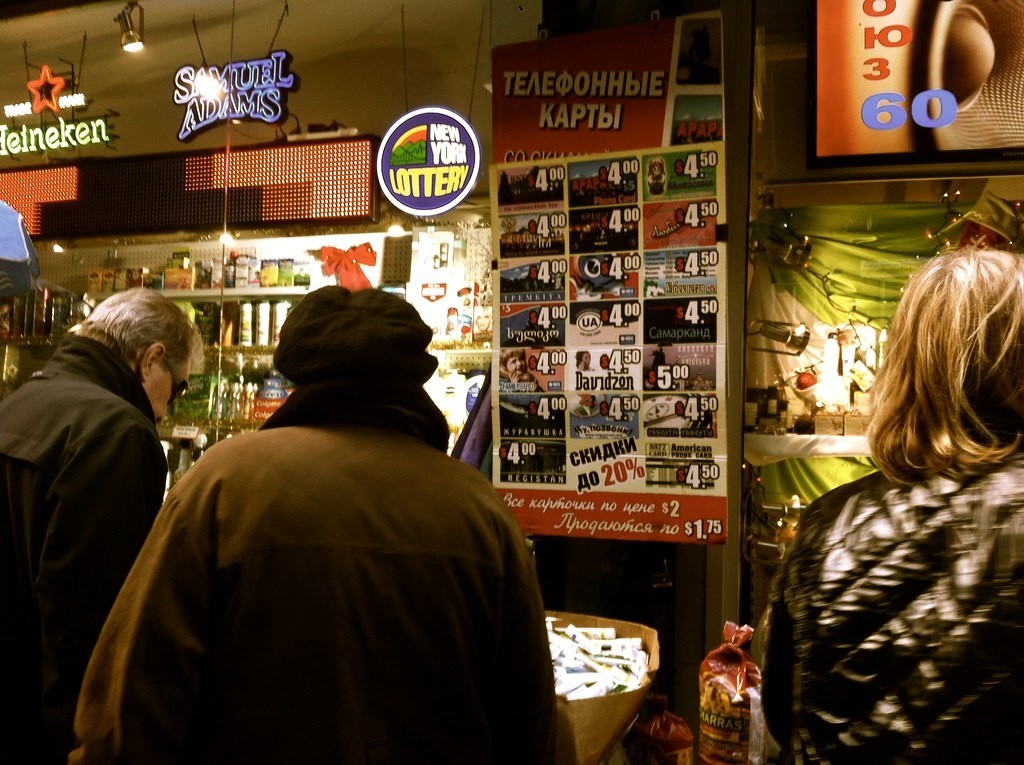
112;1;144;52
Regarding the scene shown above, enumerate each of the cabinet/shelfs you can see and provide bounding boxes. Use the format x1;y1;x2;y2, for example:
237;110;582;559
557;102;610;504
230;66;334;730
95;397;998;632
0;216;491;509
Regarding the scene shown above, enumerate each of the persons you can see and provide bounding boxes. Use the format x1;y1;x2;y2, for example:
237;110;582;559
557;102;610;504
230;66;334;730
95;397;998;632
759;245;1024;765
575;351;593;372
73;285;560;765
497;347;544;393
0;286;194;765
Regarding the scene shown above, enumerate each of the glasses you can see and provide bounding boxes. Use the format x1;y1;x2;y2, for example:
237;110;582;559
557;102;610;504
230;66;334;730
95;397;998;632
163;356;188;406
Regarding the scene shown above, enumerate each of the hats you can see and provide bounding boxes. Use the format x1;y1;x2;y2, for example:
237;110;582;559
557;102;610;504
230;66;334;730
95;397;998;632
273;286;438;386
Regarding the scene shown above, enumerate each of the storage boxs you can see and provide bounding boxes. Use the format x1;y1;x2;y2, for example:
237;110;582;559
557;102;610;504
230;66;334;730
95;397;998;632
541;607;659;765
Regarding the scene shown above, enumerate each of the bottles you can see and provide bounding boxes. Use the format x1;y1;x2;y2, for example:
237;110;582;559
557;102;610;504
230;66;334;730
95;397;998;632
86;244;311;347
744;385;793;434
168;351;294;486
0;286;96;397
